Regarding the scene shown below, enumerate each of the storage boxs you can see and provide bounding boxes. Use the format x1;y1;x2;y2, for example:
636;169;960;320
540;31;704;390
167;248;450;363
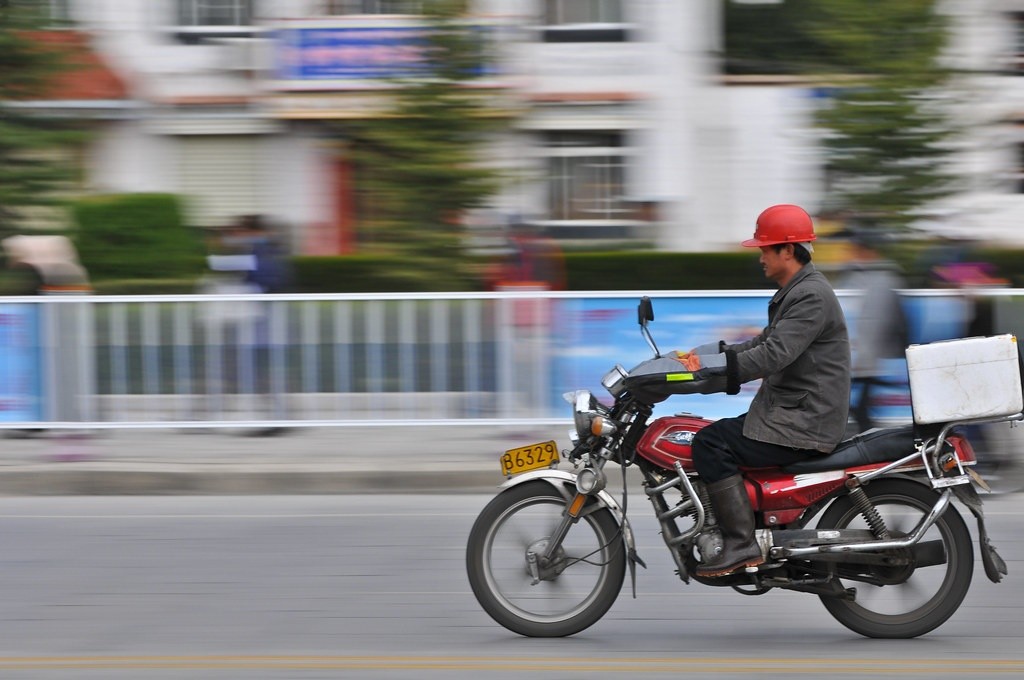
905;333;1023;426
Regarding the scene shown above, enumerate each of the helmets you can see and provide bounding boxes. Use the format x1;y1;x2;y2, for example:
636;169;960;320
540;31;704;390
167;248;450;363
741;204;816;247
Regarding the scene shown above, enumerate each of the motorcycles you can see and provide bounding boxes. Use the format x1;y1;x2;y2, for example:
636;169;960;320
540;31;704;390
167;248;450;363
465;296;1024;640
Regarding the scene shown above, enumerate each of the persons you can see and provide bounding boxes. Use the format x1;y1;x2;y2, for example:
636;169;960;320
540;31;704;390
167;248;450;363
692;205;851;578
231;212;291;435
490;218;565;430
847;242;909;404
955;286;999;464
3;234;91;437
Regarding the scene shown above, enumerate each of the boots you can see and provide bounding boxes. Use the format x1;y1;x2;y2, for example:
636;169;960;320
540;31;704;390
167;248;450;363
695;474;764;577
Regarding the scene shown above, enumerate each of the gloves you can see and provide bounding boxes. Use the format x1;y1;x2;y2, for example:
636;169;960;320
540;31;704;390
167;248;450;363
625;340;741;395
